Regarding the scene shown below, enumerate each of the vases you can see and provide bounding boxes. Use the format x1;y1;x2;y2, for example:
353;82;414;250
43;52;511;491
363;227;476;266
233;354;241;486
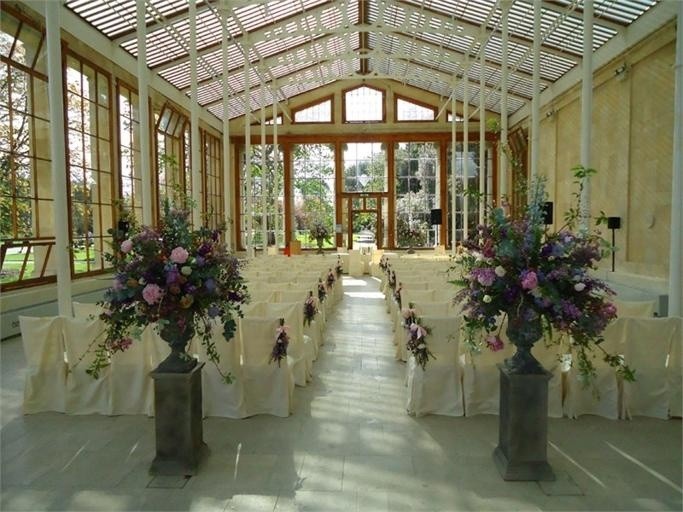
316;239;326;255
153;308;199;372
503;299;549;376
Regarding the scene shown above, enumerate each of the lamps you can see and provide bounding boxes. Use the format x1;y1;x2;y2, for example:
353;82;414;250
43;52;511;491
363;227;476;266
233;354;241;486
607;216;621;271
430;208;441;245
539;201;556;246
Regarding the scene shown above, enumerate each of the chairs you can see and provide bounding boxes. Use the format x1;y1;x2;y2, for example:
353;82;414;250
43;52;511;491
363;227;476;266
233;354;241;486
334;247;384;277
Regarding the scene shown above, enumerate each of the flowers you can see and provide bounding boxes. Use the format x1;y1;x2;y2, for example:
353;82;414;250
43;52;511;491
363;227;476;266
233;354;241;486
65;193;250;392
448;160;638;387
304;221;337;245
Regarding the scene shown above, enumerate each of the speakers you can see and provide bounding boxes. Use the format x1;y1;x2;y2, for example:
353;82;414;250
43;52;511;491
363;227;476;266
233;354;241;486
608;217;620;229
431;209;442;225
118;221;129;236
538;202;553;225
